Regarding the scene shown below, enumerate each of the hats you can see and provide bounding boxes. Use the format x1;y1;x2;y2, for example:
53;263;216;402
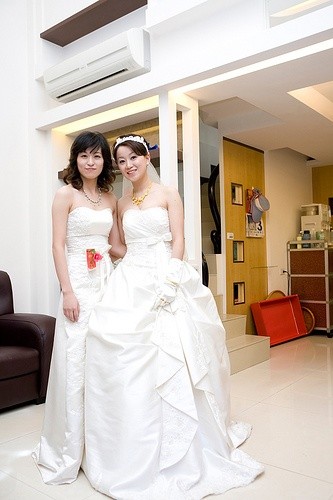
251;194;270;223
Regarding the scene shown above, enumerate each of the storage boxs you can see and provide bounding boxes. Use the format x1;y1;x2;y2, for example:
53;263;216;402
250;295;308;347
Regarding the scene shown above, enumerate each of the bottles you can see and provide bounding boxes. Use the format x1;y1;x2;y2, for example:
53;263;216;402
299;231;304;239
302;230;311;248
297;233;302;249
311;226;333;247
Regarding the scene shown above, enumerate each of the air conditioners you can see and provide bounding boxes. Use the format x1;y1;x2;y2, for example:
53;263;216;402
42;29;151;103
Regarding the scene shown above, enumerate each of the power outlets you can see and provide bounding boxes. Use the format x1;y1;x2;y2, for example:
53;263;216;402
280;268;284;274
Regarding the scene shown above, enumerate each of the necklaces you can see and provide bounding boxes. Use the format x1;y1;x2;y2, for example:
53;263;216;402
81;186;102;206
38;130;126;484
132;181;152;205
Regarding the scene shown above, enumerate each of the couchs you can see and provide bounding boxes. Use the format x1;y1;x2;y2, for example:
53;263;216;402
0;270;56;411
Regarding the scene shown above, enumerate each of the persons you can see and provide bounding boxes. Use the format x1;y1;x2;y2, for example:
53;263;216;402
80;133;263;500
